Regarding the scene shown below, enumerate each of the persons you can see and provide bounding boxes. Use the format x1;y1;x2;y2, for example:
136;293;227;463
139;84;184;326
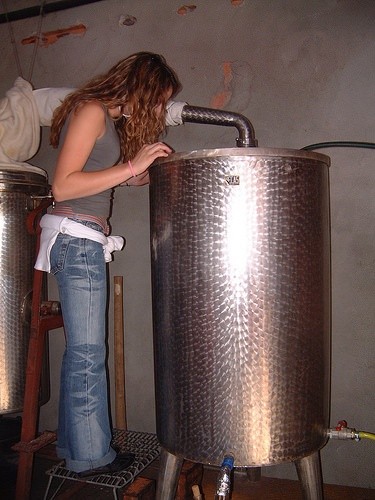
31;50;186;480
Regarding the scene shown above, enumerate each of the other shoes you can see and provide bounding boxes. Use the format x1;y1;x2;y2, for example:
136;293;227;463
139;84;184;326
111;446;121;453
76;453;135;482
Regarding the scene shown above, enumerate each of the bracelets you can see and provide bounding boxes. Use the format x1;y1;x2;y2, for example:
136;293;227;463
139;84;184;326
128;159;137;177
118;181;129;187
